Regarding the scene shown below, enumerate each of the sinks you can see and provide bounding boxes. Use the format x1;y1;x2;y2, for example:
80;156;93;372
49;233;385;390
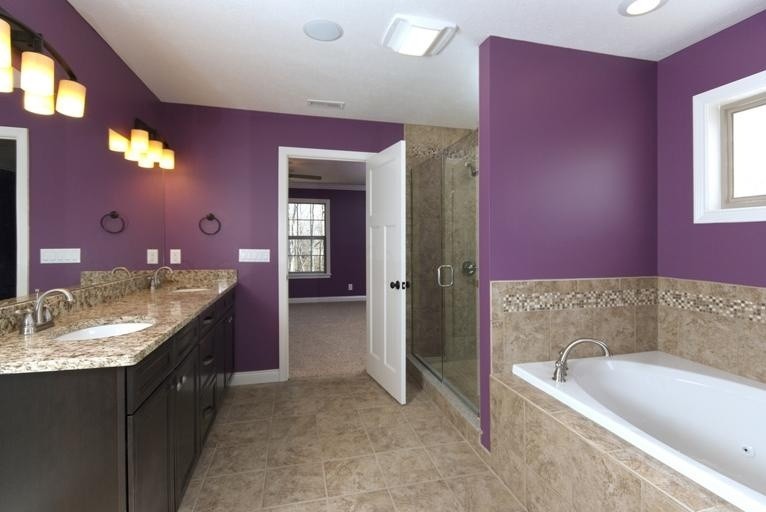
47;317;155;338
172;287;210;293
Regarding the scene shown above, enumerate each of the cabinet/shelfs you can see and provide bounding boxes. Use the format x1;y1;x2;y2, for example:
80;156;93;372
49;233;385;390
0;284;239;512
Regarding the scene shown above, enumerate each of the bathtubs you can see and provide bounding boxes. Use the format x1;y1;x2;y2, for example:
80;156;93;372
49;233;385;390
514;349;766;512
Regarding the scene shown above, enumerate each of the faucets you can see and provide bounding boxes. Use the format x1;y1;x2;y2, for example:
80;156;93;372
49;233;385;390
153;265;175;286
555;338;612;381
34;288;75;325
112;266;132;279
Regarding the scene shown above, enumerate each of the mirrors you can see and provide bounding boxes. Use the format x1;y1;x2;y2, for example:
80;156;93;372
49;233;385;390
0;0;165;310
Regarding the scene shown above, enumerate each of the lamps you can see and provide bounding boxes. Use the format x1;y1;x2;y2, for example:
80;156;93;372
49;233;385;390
0;27;56;116
381;14;458;58
1;7;89;120
131;117;177;170
107;126;156;168
